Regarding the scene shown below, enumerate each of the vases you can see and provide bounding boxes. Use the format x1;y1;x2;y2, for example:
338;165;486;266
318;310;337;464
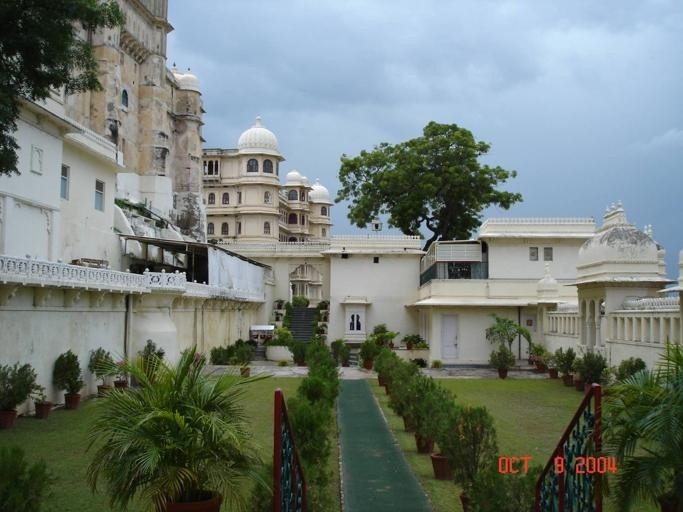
113;379;127;388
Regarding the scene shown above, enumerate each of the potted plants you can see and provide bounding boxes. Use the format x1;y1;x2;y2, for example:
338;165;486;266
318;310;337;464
0;357;41;430
528;339;607;394
288;340;342;512
374;349;441;433
592;342;682;512
235;343;254;378
88;345;114;395
52;349;84;410
403;334;429;350
339;345;350;368
359;324;401;369
34;387;52;420
430;399;537;512
485;317;531;378
